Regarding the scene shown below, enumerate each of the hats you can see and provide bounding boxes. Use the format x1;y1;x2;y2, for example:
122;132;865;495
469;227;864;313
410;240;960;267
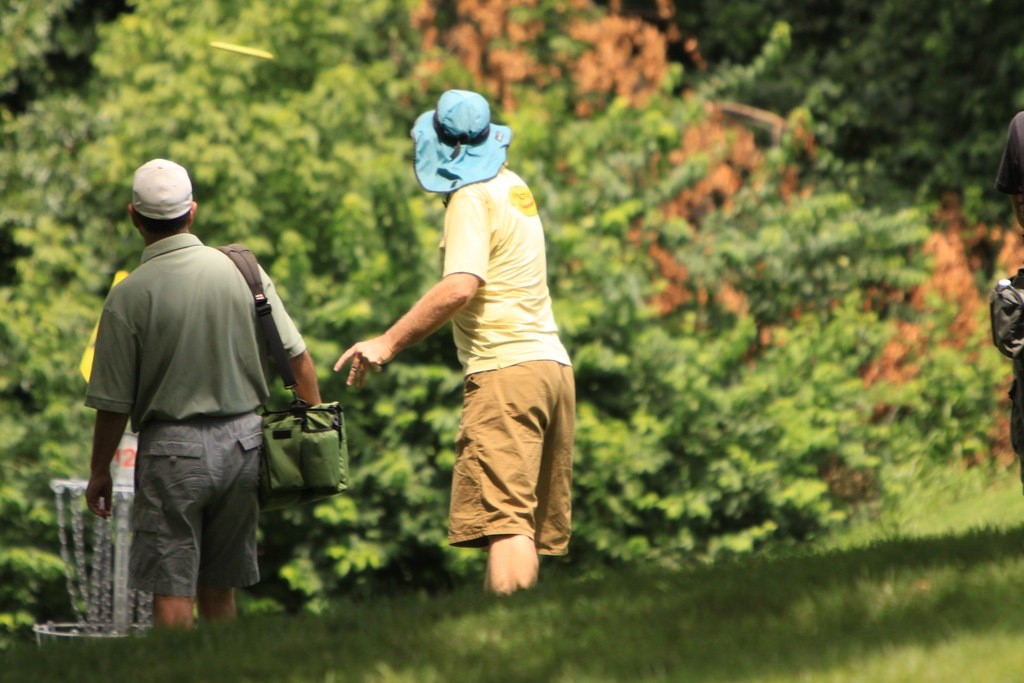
409;89;512;194
131;157;194;220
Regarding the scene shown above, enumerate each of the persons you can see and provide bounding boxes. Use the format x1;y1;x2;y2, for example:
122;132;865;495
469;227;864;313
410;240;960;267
333;89;578;596
990;109;1024;488
84;157;324;633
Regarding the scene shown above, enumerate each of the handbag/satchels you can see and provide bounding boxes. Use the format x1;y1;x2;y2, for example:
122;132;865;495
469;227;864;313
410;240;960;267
260;401;350;513
